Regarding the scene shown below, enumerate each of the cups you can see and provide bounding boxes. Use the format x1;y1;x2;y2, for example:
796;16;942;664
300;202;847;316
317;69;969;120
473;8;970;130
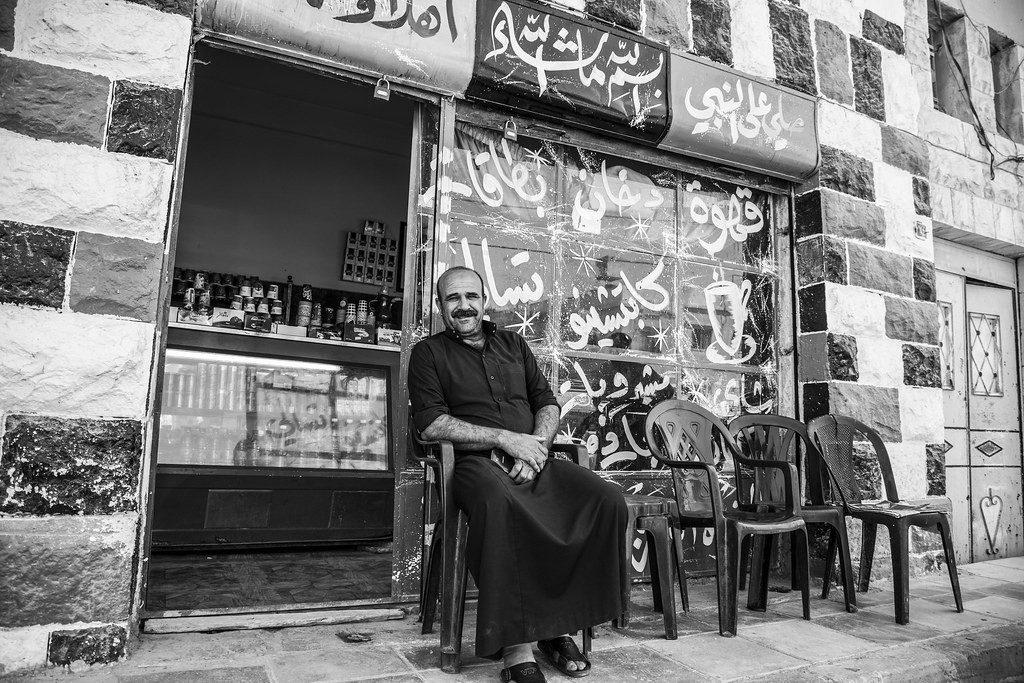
346;301;368;325
173;266;283;315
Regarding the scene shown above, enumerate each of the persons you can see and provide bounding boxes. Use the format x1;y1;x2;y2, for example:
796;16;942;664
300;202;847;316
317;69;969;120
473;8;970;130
594;334;674;418
407;267;631;683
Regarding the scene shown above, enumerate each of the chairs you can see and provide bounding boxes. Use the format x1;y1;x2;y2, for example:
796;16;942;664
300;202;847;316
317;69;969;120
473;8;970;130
728;413;859;613
805;415;963;625
405;417;595;674
645;400;813;638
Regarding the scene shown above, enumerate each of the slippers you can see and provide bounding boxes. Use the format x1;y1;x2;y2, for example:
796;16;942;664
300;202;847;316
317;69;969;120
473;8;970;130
501;662;547;683
537;635;591;676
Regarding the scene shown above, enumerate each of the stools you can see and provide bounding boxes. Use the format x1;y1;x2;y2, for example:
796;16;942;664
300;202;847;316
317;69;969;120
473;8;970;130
612;493;691;641
